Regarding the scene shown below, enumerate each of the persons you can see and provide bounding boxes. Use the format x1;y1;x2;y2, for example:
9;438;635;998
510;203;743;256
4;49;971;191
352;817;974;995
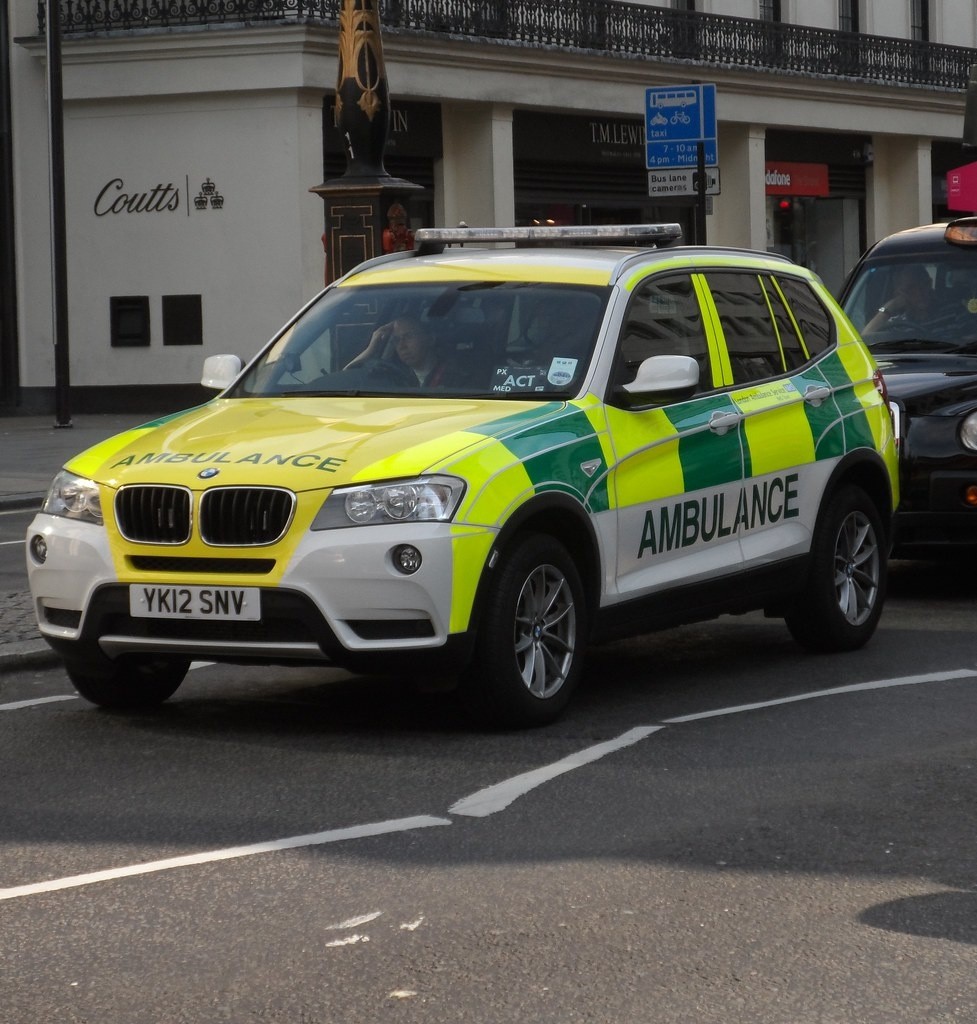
343;316;477;389
862;266;972;338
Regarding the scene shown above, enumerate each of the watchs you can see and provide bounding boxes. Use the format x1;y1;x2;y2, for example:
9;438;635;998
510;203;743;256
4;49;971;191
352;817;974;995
879;307;892;315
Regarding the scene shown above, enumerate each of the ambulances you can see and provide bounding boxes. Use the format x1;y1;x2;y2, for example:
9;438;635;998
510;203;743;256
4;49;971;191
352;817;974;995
26;223;901;729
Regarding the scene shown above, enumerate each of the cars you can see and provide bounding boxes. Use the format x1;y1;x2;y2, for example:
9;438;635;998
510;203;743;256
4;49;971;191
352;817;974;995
839;217;977;560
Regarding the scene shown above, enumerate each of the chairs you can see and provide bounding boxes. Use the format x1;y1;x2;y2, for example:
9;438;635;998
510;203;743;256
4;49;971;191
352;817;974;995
429;302;496;388
